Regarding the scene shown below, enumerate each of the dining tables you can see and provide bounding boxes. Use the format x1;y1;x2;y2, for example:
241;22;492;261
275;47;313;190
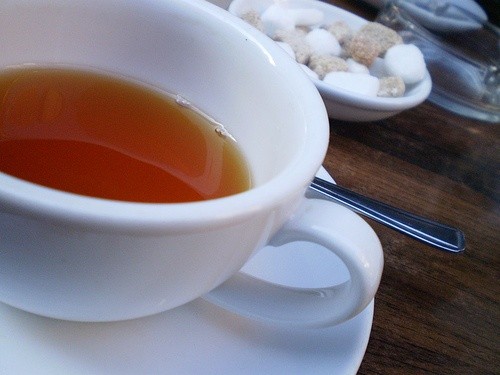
322;1;500;375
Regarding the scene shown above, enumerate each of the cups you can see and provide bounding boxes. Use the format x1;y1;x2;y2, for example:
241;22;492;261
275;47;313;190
0;0;384;327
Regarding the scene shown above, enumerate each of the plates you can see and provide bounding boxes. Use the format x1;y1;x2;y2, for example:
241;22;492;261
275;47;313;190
1;167;376;374
397;1;488;33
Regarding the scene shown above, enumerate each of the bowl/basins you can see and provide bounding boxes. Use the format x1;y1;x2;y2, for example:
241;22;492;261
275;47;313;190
375;1;499;126
227;0;433;121
